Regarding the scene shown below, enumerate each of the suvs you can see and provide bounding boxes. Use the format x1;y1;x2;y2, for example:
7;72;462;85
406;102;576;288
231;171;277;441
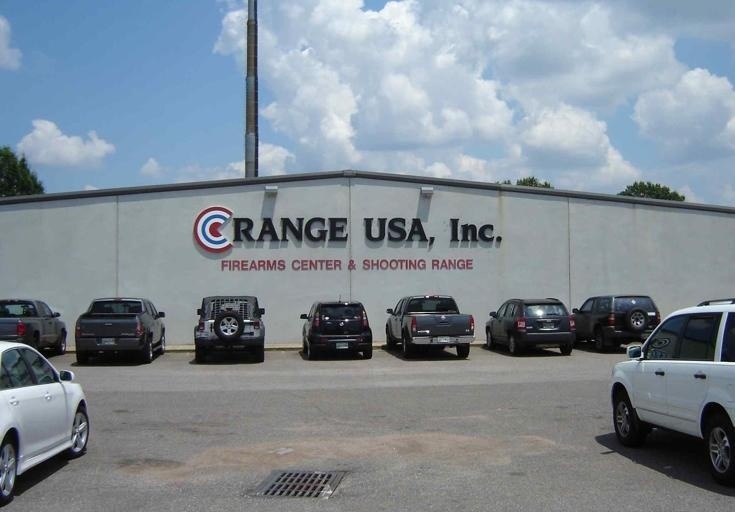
193;296;266;361
572;295;661;353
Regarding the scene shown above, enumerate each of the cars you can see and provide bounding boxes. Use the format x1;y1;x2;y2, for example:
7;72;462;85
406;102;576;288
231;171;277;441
300;295;373;359
0;298;67;355
486;298;577;356
0;341;90;506
610;297;735;489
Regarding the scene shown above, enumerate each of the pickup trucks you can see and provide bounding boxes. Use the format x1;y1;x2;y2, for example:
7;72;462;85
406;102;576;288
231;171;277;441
74;297;165;363
385;295;476;358
